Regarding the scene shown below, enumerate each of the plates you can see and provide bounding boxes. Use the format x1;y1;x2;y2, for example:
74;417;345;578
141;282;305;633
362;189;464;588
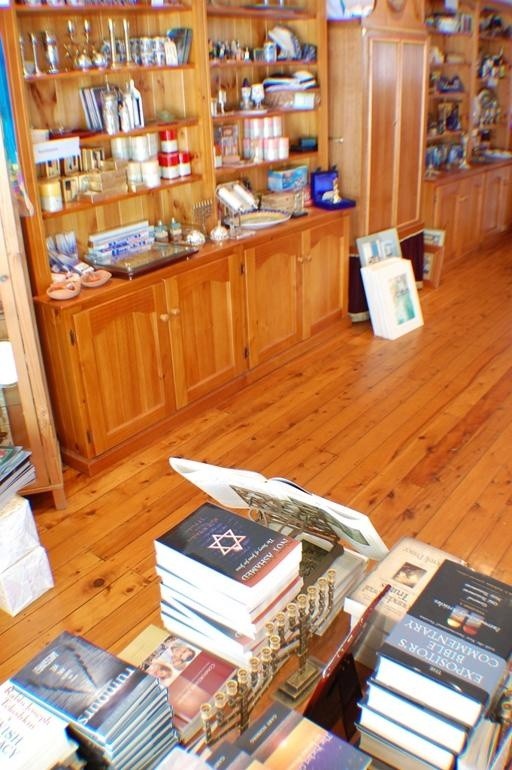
222;207;293;231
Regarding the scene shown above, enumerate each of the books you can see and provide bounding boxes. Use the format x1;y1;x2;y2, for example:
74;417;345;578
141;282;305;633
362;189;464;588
1;446;512;770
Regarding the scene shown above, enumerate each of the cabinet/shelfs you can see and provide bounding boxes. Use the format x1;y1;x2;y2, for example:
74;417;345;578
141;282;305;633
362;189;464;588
0;121;67;511
424;0;512;277
326;0;430;321
0;0;350;476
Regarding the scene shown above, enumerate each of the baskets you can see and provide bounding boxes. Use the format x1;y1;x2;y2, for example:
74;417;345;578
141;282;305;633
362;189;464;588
262;88;321;108
